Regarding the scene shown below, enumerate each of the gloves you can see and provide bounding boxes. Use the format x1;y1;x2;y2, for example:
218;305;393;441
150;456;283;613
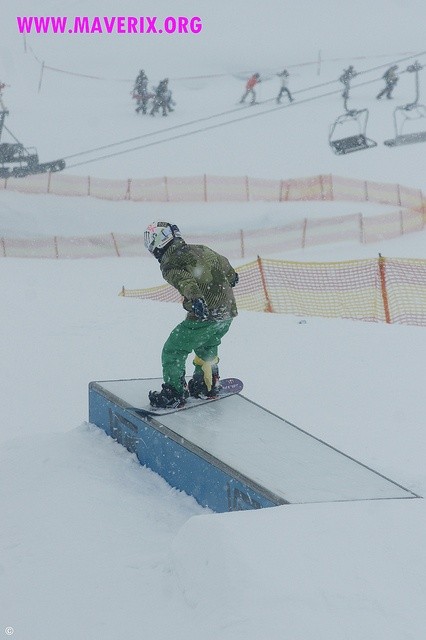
192;297;208;322
229;272;239;287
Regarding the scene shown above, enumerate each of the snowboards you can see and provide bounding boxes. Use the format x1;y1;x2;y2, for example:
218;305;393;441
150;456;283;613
125;376;243;417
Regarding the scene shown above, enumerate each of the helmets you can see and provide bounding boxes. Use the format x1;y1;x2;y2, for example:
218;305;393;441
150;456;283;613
144;221;182;259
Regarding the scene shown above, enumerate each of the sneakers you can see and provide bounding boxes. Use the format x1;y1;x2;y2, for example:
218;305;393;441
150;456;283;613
189;378;220;399
149;384;189;408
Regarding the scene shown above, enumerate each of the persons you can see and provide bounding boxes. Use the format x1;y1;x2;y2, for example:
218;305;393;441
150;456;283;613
375;64;400;101
142;219;240;409
275;69;295;105
340;65;356;99
239;72;262;104
131;68;176;117
